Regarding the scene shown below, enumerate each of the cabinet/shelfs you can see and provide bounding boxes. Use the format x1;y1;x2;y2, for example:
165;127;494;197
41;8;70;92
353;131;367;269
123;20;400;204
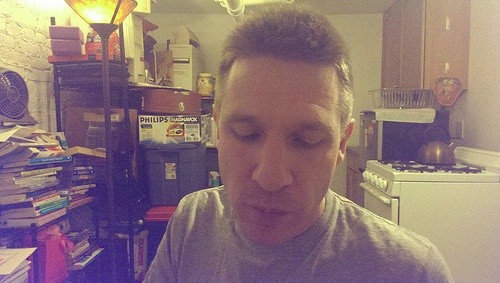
169;44;202;91
53;61;133;283
347;147;376;207
382;0;470;91
0;195;99;283
71;14;145;83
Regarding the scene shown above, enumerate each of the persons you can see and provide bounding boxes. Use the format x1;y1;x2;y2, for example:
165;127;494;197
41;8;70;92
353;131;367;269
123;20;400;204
140;0;455;283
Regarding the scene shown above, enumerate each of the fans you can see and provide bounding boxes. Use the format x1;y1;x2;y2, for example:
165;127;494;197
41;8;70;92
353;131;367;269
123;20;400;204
0;67;29;118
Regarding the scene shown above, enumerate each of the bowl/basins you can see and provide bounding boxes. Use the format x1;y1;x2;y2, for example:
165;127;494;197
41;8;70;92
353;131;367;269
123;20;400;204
368;88;434;108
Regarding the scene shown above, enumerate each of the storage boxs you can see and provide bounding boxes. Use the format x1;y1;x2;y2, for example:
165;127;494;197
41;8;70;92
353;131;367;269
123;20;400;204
138;113;212;144
49;26;84;56
144;144;207;206
368;88;436;109
144;206;177;270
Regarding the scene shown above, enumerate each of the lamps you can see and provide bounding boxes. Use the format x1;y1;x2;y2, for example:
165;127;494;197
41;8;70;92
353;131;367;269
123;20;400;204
63;0;138;283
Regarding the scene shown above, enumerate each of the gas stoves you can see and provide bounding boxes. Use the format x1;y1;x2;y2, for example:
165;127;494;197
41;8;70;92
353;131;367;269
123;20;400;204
359;160;500;283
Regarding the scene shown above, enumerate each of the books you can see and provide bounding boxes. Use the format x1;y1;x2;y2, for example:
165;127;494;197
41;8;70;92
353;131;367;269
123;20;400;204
0;125;107;283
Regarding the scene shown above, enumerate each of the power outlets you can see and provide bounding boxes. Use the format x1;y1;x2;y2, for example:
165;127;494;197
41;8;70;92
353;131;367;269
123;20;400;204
456;121;463;139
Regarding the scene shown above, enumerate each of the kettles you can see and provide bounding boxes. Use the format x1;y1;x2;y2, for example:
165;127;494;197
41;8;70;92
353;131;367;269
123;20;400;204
415;124;457;166
